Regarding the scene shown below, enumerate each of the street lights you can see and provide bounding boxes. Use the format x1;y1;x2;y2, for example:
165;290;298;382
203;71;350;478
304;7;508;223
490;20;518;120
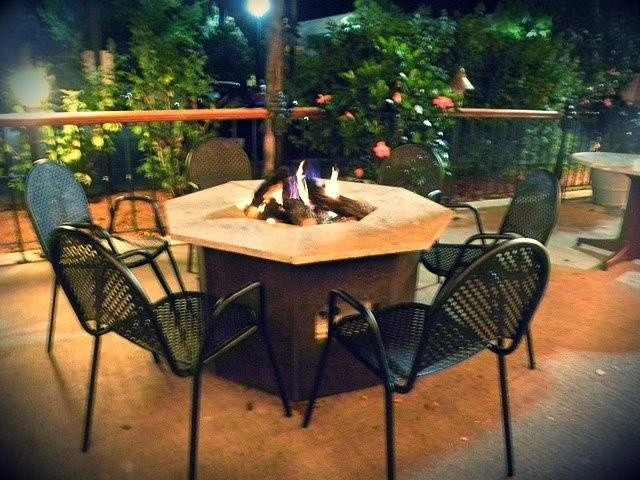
246;0;272;79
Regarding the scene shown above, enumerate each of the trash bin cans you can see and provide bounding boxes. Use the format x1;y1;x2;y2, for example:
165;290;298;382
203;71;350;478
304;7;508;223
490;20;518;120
592;168;632;210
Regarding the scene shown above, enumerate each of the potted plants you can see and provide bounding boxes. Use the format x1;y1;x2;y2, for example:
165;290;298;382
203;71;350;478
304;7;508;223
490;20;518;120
589;114;631;208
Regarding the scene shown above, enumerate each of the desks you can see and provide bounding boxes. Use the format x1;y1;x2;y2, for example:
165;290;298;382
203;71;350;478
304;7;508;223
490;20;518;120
572;152;640;270
166;177;452;402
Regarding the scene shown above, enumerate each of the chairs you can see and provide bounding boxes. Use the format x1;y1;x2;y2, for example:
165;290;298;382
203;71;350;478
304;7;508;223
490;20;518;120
302;237;549;477
45;224;293;479
423;170;559;374
379;139;444;198
185;140;254;274
25;159;195;364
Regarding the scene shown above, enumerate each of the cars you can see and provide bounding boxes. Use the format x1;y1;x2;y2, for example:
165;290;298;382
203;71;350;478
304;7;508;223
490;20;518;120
564;92;640;147
203;79;266;156
